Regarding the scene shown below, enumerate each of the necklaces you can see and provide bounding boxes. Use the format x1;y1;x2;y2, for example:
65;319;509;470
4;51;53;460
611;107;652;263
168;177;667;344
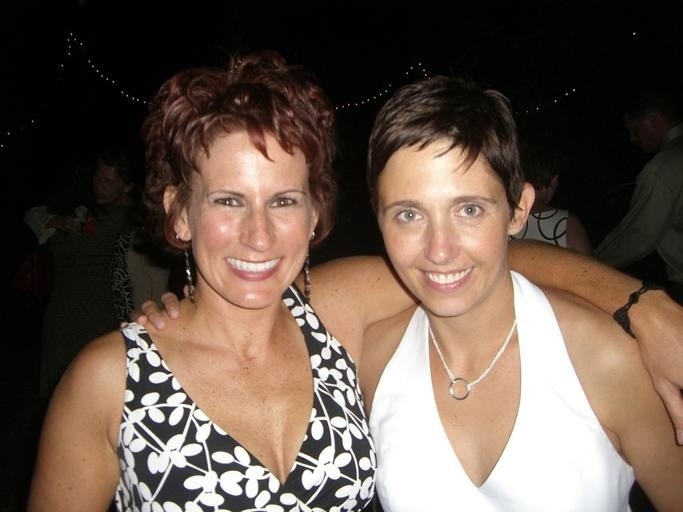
427;322;515;400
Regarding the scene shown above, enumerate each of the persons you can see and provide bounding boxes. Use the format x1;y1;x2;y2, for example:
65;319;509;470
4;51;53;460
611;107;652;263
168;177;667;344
132;76;682;511
518;135;592;254
592;87;682;306
30;56;683;511
24;151;183;410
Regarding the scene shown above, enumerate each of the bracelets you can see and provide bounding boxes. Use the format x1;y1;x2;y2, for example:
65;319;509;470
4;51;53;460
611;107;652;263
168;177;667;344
611;280;663;338
